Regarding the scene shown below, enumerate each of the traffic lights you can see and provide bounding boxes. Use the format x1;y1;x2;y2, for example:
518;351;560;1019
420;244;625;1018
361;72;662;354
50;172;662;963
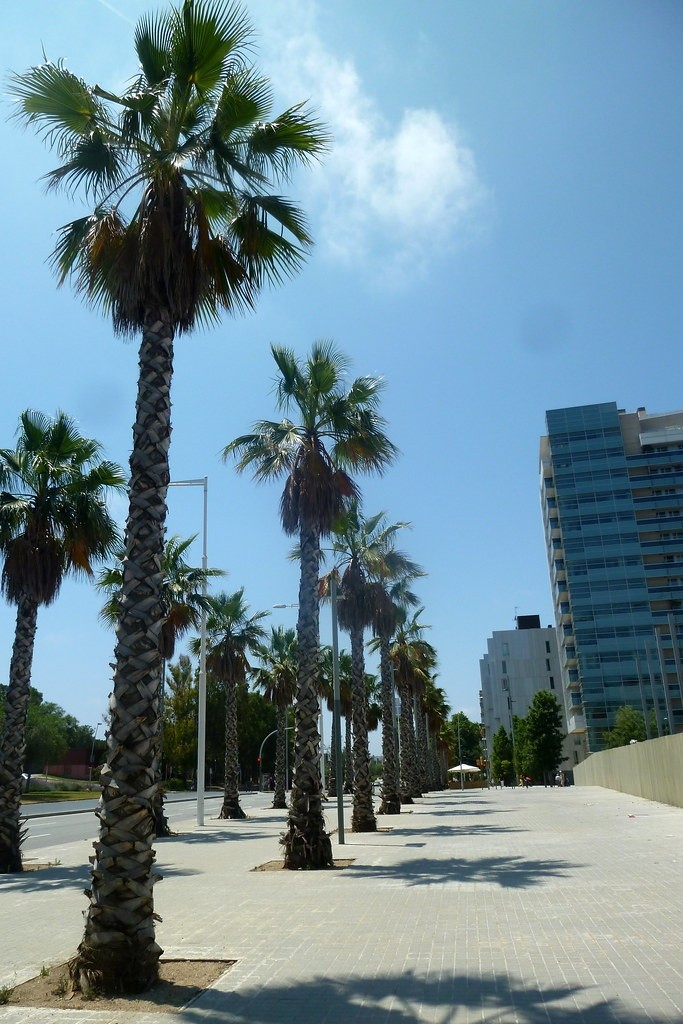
257;758;261;766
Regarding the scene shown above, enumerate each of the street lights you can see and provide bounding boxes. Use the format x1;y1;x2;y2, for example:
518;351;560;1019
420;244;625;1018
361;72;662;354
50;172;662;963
88;723;102;789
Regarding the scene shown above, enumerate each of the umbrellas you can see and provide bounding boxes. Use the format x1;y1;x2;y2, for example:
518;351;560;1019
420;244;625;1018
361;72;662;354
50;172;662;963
447;763;481;781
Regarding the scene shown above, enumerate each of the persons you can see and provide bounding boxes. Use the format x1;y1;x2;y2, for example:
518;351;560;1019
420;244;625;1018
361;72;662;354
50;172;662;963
566;777;570;786
520;774;533;788
559;770;565;787
